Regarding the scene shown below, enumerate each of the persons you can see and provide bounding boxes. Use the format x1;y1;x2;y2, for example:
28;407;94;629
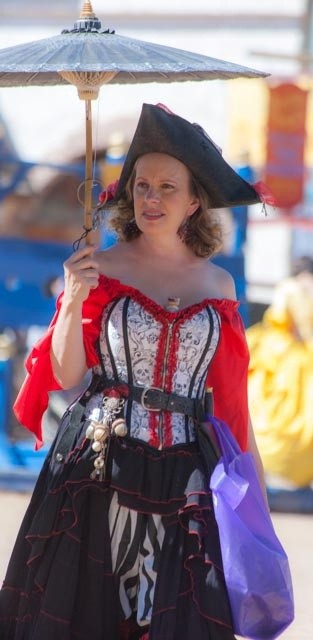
49;100;268;636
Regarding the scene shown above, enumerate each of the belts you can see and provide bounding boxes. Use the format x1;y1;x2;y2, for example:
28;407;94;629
87;375;203;419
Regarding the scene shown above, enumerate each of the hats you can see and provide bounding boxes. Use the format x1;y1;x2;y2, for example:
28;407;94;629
115;103;261;209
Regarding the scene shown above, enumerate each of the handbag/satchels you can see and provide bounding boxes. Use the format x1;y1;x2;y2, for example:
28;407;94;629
205;414;294;639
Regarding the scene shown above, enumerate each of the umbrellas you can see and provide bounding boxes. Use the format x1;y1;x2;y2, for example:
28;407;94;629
0;1;271;258
